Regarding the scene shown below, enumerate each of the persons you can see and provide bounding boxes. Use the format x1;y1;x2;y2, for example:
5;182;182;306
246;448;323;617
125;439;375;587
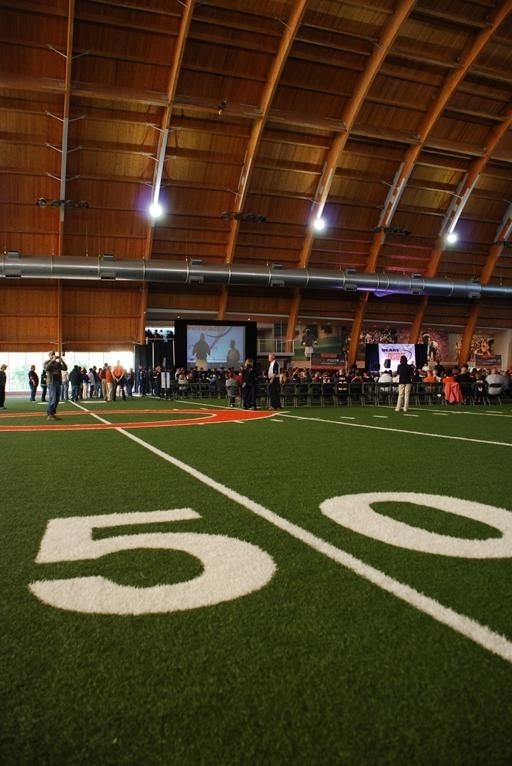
301;329;318;361
44;352;67;421
268;352;281;410
455;342;461;362
0;364;8;409
242;358;258;410
422;332;431;360
227;340;240;365
192;333;210;371
394;356;414;413
145;329;174;338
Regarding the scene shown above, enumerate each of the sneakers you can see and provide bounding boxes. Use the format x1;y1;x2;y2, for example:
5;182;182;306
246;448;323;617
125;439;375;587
47;415;58;421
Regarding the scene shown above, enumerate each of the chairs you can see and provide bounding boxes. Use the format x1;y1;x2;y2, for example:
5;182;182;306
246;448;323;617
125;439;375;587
171;381;505;409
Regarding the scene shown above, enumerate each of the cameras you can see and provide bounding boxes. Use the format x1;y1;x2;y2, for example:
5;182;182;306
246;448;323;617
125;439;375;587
56;356;59;358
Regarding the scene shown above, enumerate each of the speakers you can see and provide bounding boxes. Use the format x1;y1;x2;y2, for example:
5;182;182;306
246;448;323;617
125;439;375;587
384;359;391;368
